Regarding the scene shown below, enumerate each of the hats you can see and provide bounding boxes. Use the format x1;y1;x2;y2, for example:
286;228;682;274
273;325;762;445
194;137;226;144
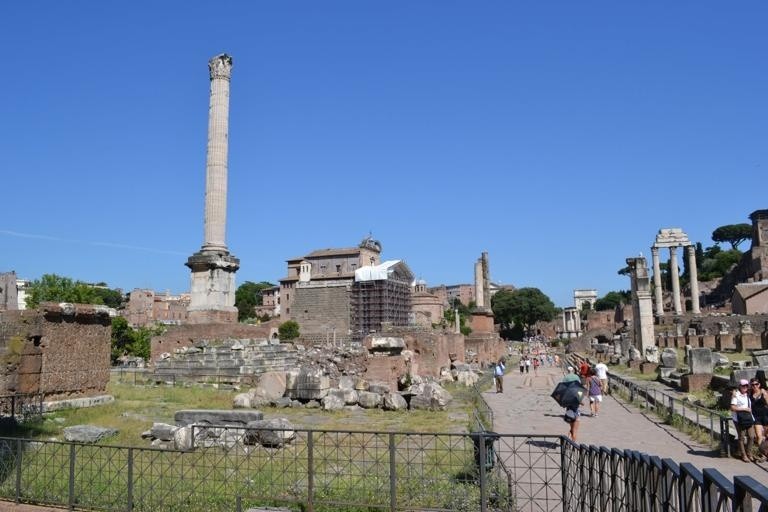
740;379;749;386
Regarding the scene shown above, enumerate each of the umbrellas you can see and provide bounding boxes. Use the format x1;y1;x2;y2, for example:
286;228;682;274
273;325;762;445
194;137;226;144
551;380;587;406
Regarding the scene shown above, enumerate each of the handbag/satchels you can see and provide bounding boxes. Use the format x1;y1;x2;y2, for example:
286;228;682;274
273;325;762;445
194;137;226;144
737;411;753;427
564;414;575;423
758;438;768;458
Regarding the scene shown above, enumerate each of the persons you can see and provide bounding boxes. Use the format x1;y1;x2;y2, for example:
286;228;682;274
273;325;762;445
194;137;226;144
564;401;585;445
580;358;609;396
562;366;582;385
519;334;559;377
730;378;768;463
589;369;603;417
493;359;506;394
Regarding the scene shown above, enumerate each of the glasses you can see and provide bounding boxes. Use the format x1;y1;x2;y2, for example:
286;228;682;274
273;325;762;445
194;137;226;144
750;383;759;387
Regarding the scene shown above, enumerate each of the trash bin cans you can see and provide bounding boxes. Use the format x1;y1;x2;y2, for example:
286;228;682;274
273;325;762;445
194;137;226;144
468;431;499;473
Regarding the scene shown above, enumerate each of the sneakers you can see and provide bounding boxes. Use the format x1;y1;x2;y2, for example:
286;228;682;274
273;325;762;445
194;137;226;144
591;413;598;417
